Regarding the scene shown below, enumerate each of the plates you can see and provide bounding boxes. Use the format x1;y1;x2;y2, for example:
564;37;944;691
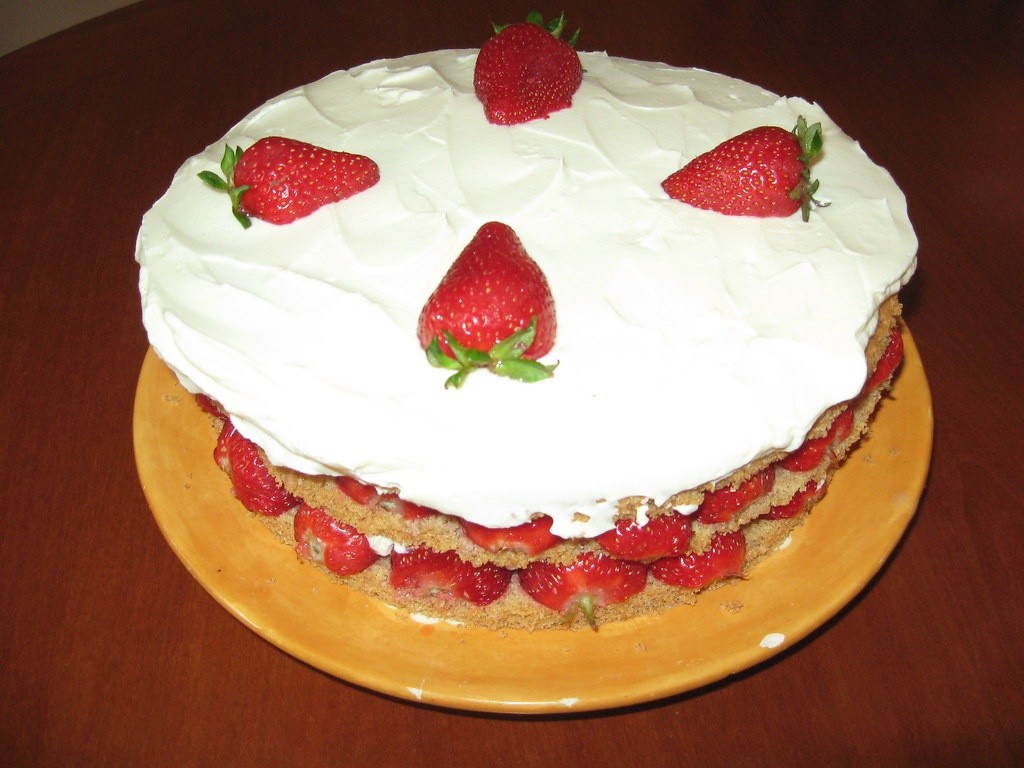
131;325;936;716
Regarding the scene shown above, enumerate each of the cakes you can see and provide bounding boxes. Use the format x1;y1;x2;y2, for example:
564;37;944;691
133;7;920;629
